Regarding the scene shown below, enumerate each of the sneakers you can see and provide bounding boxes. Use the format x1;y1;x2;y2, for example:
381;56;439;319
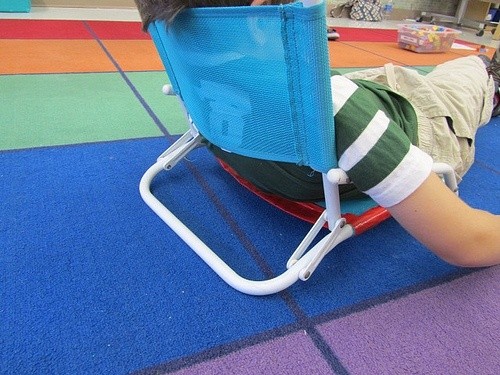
484;46;500;88
492;90;500;118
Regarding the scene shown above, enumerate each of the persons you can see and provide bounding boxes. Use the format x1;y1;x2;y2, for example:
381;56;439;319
140;0;500;268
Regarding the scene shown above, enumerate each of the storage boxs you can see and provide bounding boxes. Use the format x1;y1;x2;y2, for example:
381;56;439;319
395;23;463;54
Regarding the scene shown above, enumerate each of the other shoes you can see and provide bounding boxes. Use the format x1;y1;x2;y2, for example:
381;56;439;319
327;26;340;40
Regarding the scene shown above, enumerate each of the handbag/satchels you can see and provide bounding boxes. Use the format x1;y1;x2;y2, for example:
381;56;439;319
350;0;383;22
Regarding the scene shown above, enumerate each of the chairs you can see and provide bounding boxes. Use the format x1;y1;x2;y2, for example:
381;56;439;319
137;0;391;298
